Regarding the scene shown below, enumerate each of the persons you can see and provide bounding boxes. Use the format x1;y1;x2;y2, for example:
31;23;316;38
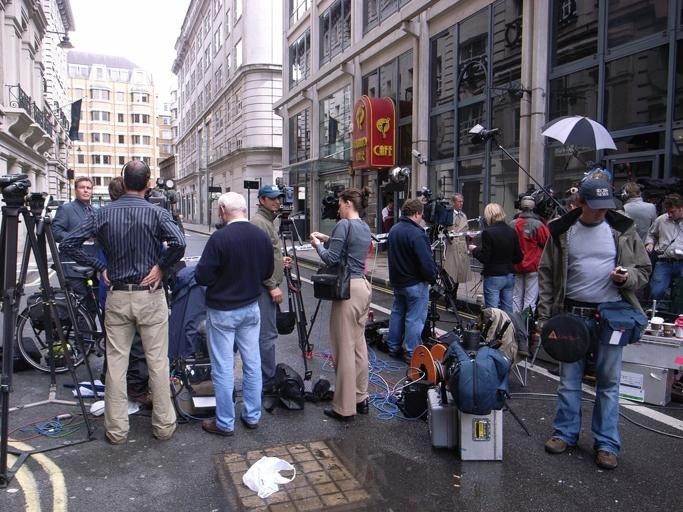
536;178;652;469
107;176;126;200
247;184;293;393
385;198;438;365
616;181;657;239
468;202;524;315
52;177;101;364
508;195;550;315
441;193;472;314
311;187;373;422
382;199;395;233
59;160;187;445
645;194;683;316
194;192;275;437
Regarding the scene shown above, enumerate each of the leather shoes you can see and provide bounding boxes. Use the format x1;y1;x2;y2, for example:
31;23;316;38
356;399;369;414
324;408;353;422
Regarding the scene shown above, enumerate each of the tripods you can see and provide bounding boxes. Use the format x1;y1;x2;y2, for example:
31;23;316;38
16;214;101;408
281;232;314;380
0;205;99;488
428;227;462;336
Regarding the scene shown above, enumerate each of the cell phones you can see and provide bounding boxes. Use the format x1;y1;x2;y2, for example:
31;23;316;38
616;268;628;274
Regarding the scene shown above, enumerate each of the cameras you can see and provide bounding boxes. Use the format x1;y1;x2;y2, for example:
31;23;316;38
0;174;31;205
147;178;180;225
274;185;303;246
415;187;458;245
26;192;53;218
321;181;345;221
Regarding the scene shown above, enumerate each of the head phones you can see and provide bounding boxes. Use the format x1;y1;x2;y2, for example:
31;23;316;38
121;160;151;192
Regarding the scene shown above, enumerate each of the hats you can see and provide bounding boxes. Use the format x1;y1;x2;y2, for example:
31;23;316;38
258;184;286;199
580;178;616;210
521;196;536;210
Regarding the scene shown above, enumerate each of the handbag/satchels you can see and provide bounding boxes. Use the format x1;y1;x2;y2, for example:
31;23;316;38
261;363;305;413
595;301;649;348
310;217;350;301
540;315;593;363
276;269;296;335
443;342;511;415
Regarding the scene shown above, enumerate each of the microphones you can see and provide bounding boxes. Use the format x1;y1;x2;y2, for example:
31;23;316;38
480;128;499;136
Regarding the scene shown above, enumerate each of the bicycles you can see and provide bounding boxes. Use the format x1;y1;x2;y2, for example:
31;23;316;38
15;265;172;374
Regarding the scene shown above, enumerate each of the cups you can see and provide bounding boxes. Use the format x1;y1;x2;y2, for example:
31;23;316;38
664;323;675;337
650;321;662;329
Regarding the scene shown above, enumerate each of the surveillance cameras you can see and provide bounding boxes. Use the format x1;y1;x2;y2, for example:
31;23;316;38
412;149;421;158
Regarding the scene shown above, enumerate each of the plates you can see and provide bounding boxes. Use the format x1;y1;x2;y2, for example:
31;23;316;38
646;329;662;336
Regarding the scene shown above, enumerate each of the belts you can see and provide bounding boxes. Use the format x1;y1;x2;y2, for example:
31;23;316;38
564;299;596;316
107;284;164;291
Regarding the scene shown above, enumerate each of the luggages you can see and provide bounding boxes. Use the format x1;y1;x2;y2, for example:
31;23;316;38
426;387;456;449
456;410;503;462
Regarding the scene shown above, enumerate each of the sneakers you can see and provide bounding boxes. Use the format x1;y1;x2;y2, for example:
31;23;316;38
544;438;570;454
242;421;259;430
595;450;619;469
202;420;234;437
447;307;456;313
